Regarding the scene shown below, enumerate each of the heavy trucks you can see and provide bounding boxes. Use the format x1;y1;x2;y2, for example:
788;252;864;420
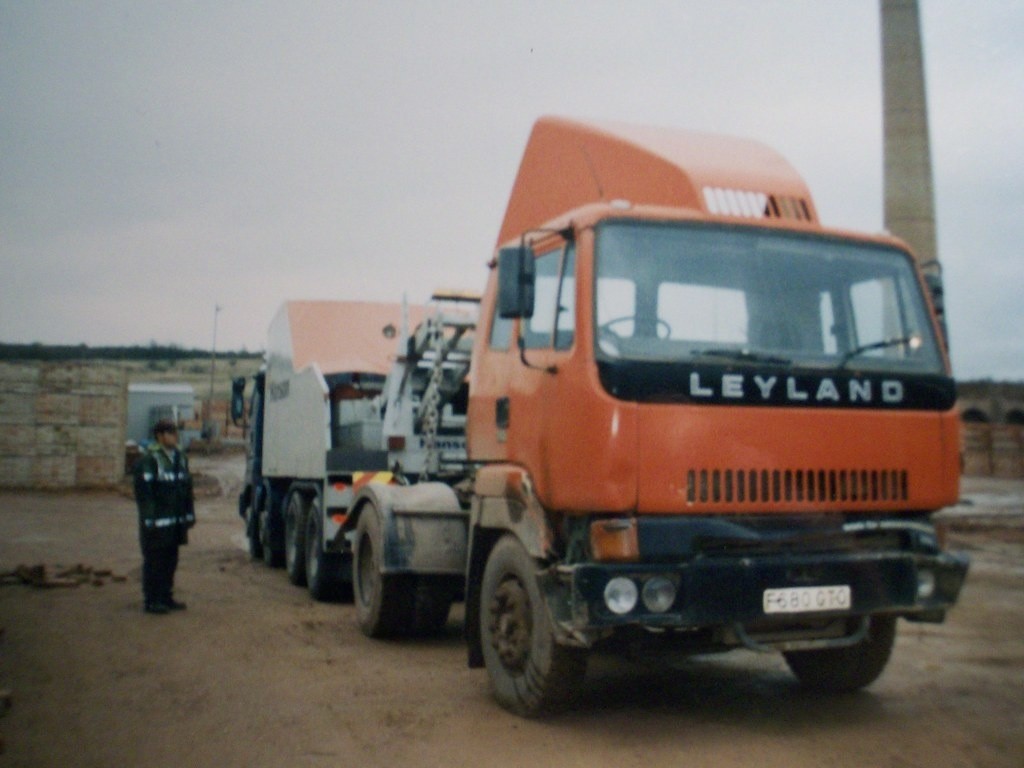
238;114;971;715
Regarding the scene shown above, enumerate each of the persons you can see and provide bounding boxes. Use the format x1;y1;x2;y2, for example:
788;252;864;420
132;418;198;614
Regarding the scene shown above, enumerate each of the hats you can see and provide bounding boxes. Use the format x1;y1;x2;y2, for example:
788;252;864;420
154;419;178;433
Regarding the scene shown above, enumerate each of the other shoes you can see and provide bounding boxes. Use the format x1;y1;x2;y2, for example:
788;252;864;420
146;600;169;614
165;599;187;610
